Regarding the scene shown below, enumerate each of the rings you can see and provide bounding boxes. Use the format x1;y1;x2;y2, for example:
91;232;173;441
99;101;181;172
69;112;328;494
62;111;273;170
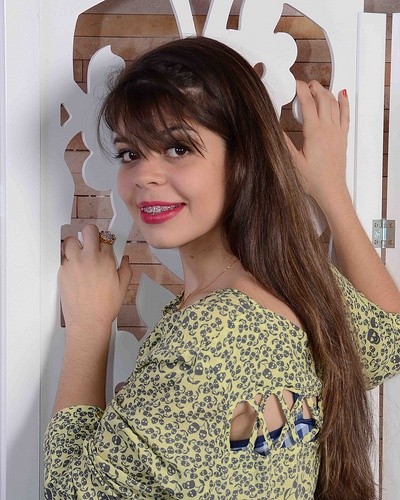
98;230;117;245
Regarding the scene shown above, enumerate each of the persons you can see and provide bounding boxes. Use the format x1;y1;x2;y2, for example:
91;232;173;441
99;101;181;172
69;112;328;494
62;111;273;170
40;34;400;500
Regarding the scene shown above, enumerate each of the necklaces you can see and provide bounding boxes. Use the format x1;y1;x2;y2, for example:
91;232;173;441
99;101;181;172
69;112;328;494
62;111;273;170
177;256;240;311
